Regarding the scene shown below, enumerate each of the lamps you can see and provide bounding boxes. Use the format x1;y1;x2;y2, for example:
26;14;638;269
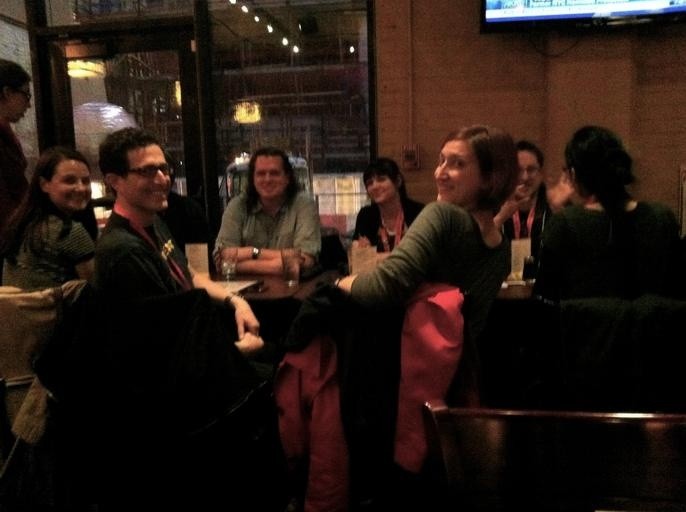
231;97;261;124
67;59;106;79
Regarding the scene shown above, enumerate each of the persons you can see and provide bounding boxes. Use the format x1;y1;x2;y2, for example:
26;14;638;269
214;147;322;282
333;124;520;392
0;146;98;294
158;151;214;259
494;137;576;281
86;127;266;362
537;125;686;298
0;56;33;222
351;161;425;253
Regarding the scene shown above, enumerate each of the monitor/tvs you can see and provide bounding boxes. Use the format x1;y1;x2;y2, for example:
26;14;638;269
482;0;685;25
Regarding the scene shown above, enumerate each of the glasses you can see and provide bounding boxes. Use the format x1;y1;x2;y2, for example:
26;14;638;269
518;165;542;177
12;86;33;101
127;164;170;178
561;163;576;173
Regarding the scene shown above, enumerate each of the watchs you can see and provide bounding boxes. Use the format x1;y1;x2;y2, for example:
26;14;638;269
223;291;236;306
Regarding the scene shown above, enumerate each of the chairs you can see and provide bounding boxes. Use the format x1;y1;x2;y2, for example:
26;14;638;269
420;397;683;511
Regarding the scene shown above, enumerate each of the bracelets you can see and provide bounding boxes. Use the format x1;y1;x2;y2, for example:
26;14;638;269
252;247;260;260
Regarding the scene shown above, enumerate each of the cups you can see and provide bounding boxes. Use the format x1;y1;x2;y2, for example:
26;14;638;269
280;246;302;290
220;241;238;281
